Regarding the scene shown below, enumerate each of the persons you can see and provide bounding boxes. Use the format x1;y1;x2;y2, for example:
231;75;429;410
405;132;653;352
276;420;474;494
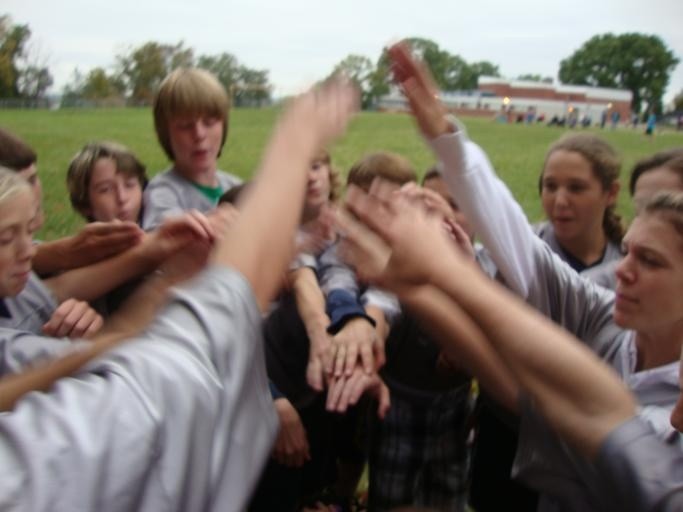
1;42;683;511
